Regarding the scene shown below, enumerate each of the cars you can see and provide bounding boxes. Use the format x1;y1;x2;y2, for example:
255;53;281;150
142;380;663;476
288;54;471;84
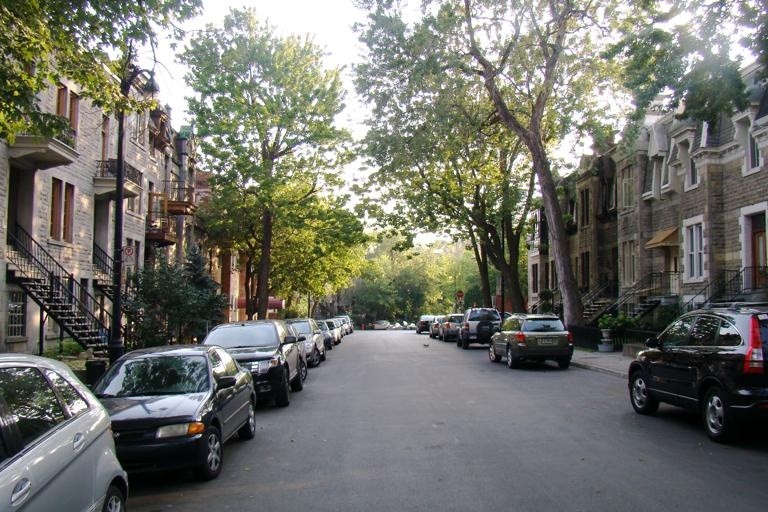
416;313;464;341
374;319;415;330
316;315;353;349
93;343;257;478
0;352;128;512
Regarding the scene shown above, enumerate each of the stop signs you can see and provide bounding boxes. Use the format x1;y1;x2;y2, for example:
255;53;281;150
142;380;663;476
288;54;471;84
456;290;464;298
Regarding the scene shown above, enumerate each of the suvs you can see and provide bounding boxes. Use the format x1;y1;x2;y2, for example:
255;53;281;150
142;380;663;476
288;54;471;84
488;312;574;368
458;306;503;348
202;318;325;406
628;306;768;439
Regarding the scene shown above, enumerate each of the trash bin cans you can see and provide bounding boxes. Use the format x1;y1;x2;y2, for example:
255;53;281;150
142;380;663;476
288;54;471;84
85;361;105;386
361;323;365;330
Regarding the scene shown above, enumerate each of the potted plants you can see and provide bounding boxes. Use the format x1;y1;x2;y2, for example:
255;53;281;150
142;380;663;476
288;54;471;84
598;313;619;338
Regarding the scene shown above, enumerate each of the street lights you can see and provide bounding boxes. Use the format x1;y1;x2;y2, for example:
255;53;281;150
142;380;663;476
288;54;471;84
109;68;158;359
